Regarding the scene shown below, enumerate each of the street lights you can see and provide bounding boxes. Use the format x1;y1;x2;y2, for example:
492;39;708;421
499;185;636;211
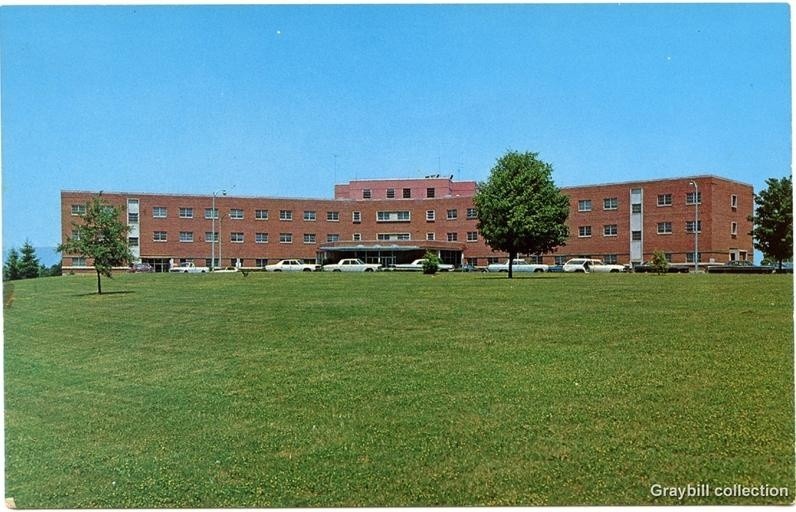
689;179;699;272
211;187;228;268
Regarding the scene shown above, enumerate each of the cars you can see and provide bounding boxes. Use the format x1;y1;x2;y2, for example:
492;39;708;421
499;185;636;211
563;258;629;272
213;266;239;272
265;259;322;272
130;263;154;273
726;260;756;267
488;259;549;272
324;258;382;272
391;258;454;271
769;262;788;267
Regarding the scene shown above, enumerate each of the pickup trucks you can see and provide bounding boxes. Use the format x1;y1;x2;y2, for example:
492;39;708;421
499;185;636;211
170;261;209;273
